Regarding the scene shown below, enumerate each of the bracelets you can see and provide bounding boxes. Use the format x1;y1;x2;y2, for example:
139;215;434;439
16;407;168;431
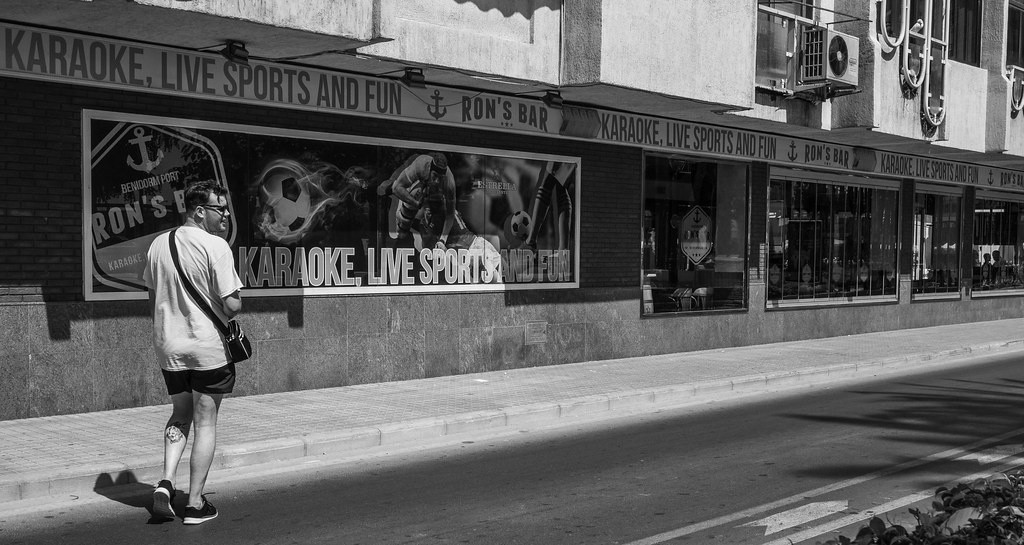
415;201;419;208
438;239;445;245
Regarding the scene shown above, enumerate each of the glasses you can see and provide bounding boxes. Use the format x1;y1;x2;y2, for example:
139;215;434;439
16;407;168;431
200;205;230;212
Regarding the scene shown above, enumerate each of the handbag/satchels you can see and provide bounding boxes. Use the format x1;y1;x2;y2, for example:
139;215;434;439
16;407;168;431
226;320;252;361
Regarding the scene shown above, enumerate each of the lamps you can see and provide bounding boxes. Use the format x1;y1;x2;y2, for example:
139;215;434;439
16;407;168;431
216;39;250;68
541;89;564;109
399;66;427;90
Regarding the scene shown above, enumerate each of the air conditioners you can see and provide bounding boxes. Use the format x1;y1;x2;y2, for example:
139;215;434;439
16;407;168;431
800;24;860;87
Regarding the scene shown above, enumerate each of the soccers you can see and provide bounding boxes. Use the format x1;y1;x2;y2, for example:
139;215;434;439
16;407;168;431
256;167;311;235
510;211;532;238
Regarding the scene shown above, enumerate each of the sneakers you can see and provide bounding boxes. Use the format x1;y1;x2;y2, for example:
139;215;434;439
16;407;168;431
153;480;176;517
185;492;218;524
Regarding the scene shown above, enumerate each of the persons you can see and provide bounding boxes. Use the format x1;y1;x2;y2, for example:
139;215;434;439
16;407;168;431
142;180;244;524
516;161;575;257
392;153;456;253
982;250;1007;268
